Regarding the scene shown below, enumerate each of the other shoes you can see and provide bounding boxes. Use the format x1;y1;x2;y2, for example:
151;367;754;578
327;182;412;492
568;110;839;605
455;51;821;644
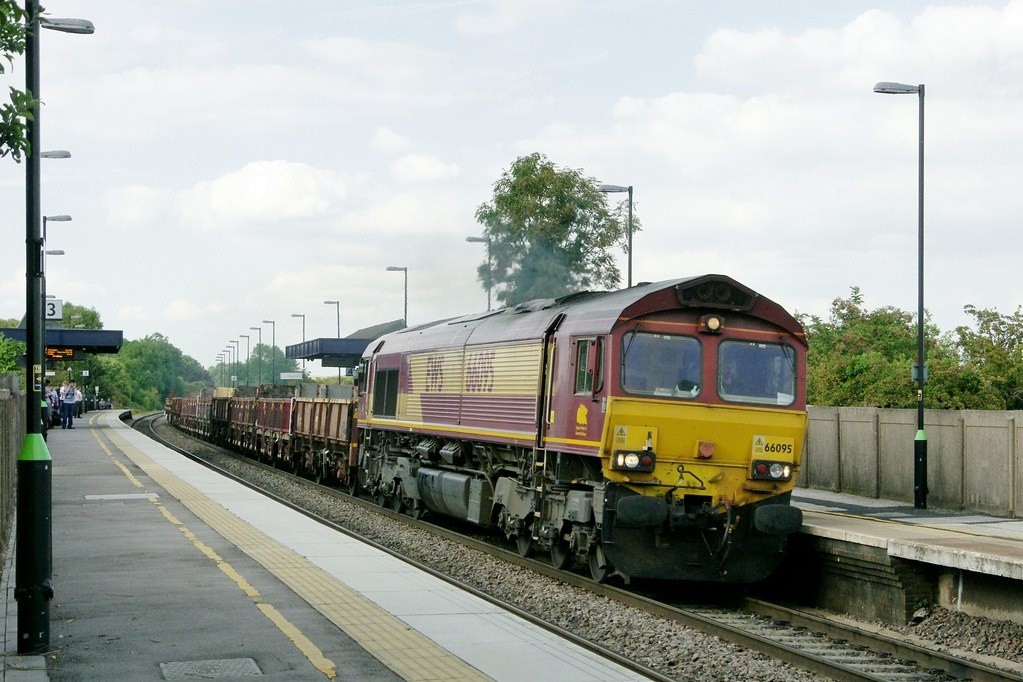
67;426;75;429
78;416;82;418
62;425;66;429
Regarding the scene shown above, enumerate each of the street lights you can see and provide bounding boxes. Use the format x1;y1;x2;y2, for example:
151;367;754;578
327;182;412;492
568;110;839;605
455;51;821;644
466;236;491;311
239;335;250;382
226;345;235;388
324;300;340;385
11;17;94;654
263;320;275;383
873;79;930;509
218;353;226;386
223;350;230;387
600;184;633;288
230;341;239;386
250;327;261;384
387;266;408;329
216;357;223;387
291;314;305;382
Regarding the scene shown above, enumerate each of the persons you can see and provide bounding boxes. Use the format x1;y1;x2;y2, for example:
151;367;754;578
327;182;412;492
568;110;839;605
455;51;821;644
74;386;82;418
49;384;59;411
59;381;69;408
55;383;61;398
99;399;113;410
62;380;77;429
45;380;52;421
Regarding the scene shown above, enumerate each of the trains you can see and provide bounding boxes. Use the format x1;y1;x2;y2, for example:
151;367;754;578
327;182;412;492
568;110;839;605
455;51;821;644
165;275;808;585
